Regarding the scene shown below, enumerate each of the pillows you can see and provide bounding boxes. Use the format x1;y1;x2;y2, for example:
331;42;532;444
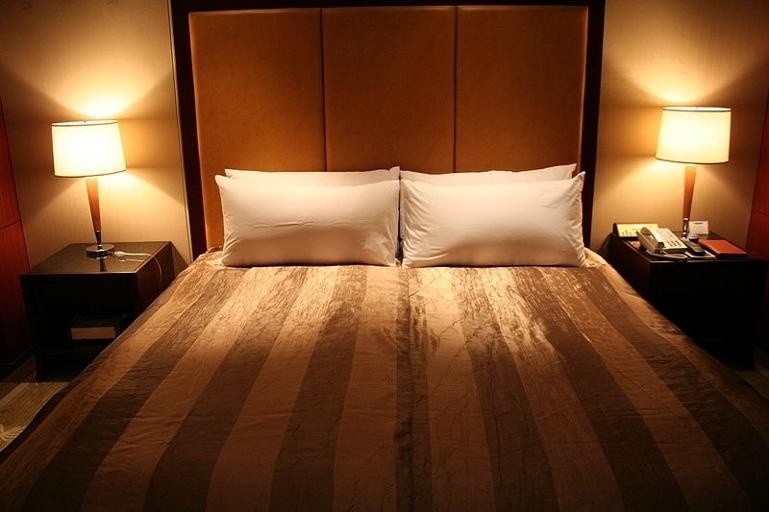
400;163;578;186
212;168;400;266
224;166;401;186
401;171;587;269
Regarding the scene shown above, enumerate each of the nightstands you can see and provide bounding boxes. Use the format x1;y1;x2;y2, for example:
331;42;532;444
18;243;177;380
613;231;767;374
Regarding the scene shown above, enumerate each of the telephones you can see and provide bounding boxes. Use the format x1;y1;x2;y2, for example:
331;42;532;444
636;225;687;254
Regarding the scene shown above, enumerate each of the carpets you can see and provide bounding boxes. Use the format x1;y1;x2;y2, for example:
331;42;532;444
0;367;69;453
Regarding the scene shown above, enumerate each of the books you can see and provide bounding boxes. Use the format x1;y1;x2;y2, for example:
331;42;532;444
697;239;747;259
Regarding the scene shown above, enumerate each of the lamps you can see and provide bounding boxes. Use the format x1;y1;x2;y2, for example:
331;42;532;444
49;120;129;258
654;106;731;237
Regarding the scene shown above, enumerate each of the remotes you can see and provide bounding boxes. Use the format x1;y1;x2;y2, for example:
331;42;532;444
681;239;705;255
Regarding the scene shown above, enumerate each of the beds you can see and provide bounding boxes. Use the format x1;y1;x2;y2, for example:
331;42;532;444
5;247;768;512
